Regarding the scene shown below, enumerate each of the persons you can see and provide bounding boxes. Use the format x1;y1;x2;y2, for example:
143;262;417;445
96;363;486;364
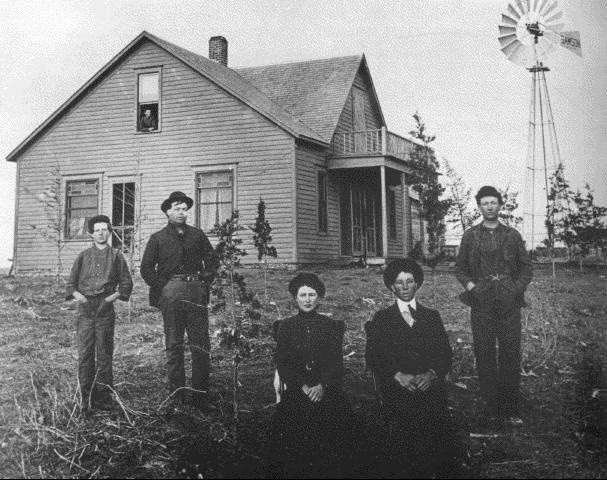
139;192;215;409
264;273;346;480
140;110;157;132
364;258;453;480
453;186;533;428
64;215;133;409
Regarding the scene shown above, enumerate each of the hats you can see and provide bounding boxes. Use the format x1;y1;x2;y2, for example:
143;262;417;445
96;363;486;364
160;191;193;213
287;273;325;297
382;257;425;292
